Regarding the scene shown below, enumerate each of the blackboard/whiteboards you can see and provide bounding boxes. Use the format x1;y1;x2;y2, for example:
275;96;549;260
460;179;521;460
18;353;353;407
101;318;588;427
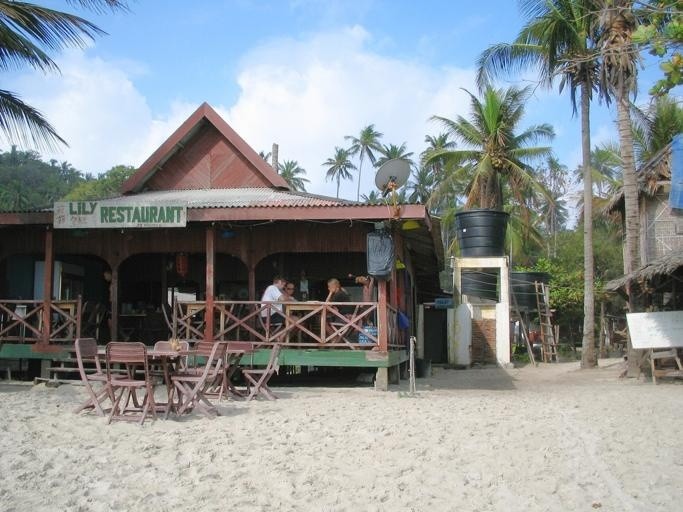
625;310;683;349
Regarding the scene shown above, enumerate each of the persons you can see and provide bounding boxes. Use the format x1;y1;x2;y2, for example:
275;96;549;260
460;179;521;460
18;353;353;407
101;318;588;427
260;274;301;348
326;277;356;343
284;280;299;301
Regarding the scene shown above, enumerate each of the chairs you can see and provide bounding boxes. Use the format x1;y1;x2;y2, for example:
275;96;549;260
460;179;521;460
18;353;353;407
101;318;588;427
36;302;370;425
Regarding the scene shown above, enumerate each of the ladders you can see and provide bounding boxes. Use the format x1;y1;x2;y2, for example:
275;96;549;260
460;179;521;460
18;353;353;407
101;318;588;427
535;281;559;364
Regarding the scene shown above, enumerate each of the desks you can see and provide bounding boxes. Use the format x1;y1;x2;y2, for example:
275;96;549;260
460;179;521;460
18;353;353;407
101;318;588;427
70;349;200;413
285;302;328;348
38;302;75;344
177;349;245;399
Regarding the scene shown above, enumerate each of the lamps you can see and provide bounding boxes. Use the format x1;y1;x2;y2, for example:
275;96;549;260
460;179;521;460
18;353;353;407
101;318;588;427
402;221;420;230
396;259;405;269
